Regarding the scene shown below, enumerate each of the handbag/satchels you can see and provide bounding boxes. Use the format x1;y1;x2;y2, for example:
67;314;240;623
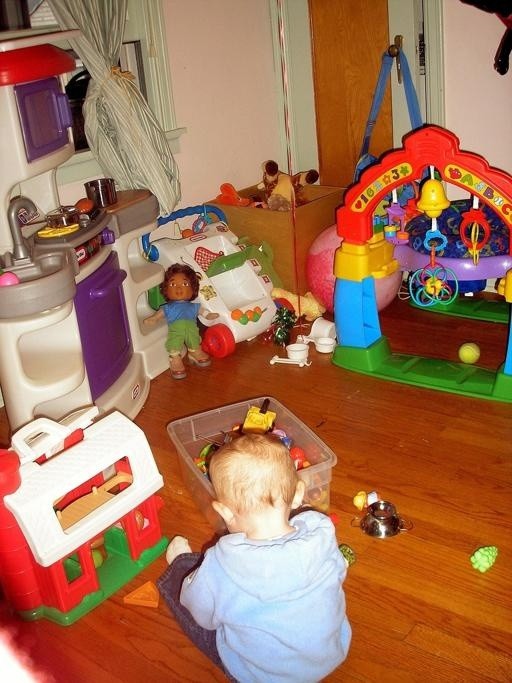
353;153;431;208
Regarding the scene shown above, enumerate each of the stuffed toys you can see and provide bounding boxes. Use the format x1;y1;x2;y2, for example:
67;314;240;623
254;160;318;212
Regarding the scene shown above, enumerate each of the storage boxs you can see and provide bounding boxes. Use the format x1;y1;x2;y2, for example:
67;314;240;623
204;180;348;296
166;396;338;537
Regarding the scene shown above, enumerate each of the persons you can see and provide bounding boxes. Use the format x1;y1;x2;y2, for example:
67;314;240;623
157;434;352;683
143;264;219;380
